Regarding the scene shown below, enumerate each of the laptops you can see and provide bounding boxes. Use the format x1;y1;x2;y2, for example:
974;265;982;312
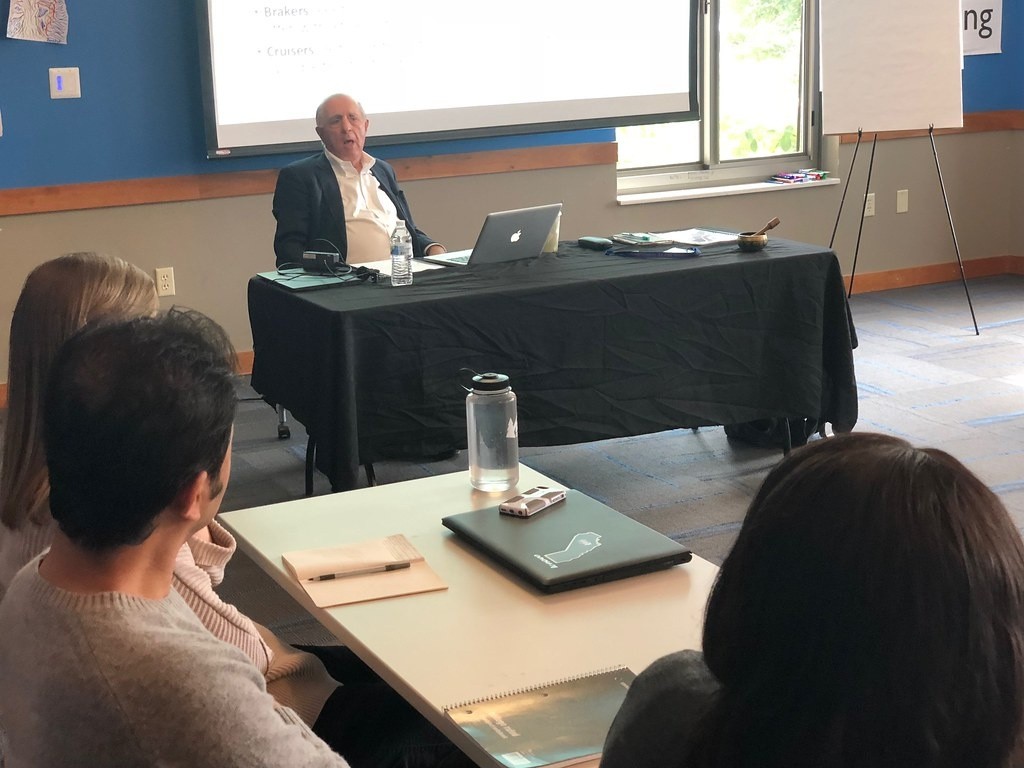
424;203;562;267
443;488;693;594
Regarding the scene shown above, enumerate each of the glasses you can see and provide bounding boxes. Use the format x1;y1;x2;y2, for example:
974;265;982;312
323;115;361;128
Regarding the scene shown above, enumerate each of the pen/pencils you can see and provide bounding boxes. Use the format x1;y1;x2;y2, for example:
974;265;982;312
307;562;413;583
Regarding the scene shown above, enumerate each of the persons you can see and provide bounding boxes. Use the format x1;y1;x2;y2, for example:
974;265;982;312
0;252;478;768
273;93;445;266
601;432;1024;768
0;309;350;768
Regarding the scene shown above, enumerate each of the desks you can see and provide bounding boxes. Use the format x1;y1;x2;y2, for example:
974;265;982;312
247;227;860;499
216;461;721;768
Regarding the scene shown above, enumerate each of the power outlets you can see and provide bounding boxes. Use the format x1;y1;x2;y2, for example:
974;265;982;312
863;193;875;218
154;267;174;297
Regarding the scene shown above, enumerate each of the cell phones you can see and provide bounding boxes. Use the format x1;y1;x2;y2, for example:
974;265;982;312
498;486;566;517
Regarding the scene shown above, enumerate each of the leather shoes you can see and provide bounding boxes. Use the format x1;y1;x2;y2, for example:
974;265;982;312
427;449;461;461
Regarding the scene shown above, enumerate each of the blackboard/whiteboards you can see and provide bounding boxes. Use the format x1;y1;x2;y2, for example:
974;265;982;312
819;0;965;137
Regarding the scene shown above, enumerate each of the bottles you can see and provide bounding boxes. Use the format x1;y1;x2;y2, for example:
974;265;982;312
456;367;520;492
390;220;413;287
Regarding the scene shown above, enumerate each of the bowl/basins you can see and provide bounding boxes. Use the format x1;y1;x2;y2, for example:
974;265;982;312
738;231;768;252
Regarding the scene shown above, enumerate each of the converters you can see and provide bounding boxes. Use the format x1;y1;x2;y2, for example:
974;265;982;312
303;251;339;274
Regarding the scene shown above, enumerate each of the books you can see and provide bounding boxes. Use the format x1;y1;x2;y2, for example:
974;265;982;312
443;665;637;768
281;533;448;608
